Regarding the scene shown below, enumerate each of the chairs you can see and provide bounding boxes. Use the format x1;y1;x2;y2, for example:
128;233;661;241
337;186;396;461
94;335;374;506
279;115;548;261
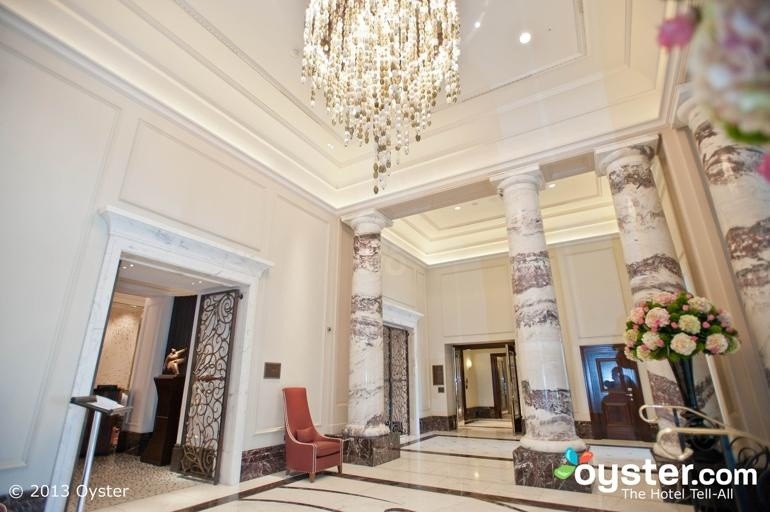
277;383;344;483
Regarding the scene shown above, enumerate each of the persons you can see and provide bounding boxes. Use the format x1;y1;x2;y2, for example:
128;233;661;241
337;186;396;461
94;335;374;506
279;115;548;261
162;346;188;374
602;366;637;399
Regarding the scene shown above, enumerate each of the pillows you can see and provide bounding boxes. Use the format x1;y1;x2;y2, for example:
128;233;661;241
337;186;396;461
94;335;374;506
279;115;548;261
295;424;318;444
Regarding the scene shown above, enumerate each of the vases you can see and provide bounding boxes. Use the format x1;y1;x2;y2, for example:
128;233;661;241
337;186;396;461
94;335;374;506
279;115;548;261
664;354;739;509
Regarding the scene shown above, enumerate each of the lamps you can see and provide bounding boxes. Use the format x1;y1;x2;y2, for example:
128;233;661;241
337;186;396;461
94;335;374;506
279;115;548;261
298;0;468;199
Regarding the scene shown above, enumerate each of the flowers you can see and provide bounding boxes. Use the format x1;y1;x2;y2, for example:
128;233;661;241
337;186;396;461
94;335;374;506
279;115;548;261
622;285;739;364
